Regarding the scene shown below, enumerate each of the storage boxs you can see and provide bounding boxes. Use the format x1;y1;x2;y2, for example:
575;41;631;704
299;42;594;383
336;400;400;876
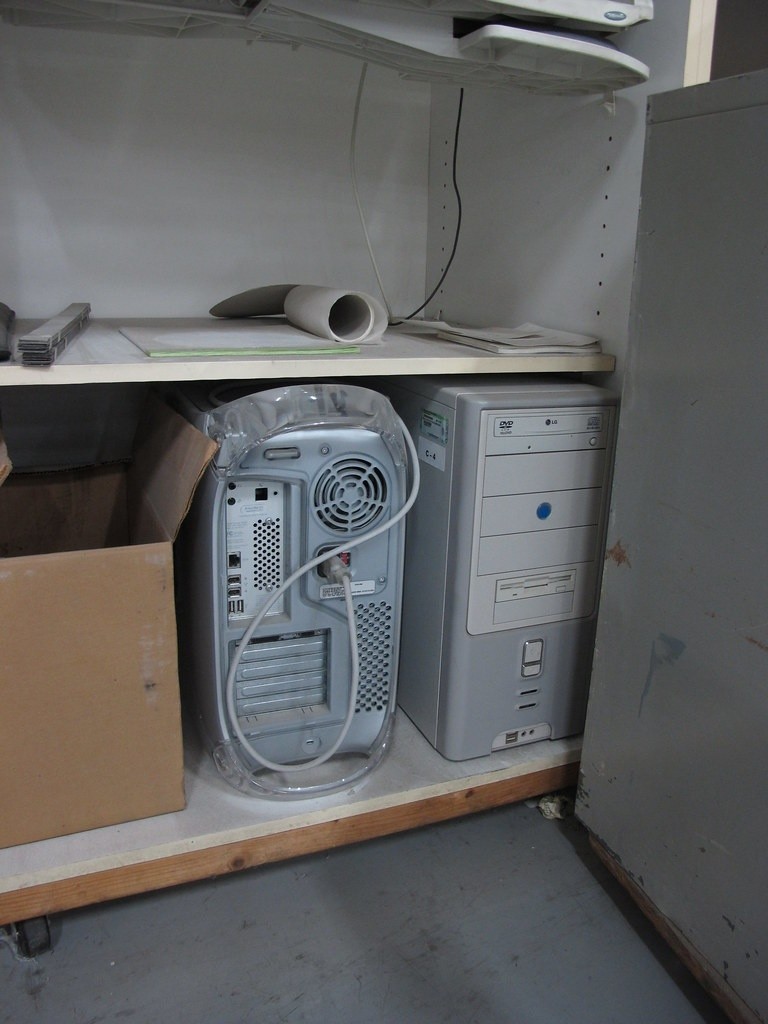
0;403;218;853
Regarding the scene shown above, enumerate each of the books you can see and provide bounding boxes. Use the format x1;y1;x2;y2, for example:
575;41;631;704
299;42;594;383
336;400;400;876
437;330;602;353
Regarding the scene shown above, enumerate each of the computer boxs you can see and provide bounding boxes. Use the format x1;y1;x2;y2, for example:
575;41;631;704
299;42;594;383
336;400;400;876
167;385;406;800
391;378;621;762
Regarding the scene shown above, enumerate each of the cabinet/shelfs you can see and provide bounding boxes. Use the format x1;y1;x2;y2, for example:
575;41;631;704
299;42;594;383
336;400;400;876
0;320;622;928
